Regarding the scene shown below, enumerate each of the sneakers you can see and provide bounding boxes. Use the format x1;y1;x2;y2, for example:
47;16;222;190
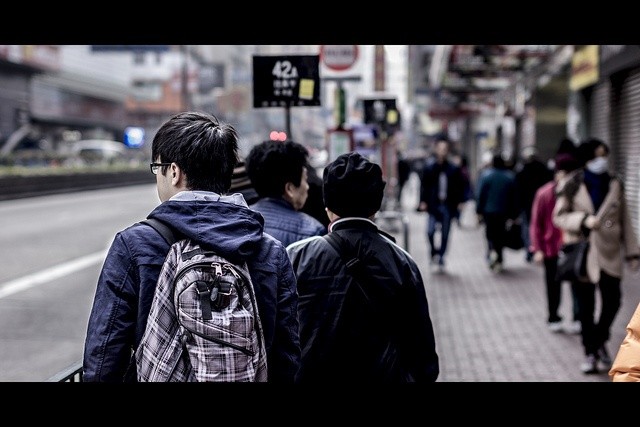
598;346;612;370
583;352;596;371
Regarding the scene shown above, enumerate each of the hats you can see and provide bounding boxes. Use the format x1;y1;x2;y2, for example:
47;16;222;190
547;138;574;170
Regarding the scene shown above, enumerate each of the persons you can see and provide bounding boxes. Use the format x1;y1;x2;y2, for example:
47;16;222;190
608;302;640;386
77;109;302;396
475;152;516;272
527;137;589;324
551;137;640;373
420;135;467;275
286;153;439;394
393;158;421;253
446;149;470;228
246;138;326;250
512;144;552;260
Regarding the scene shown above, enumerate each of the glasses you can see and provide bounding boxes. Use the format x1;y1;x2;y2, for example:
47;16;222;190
151;162;171;174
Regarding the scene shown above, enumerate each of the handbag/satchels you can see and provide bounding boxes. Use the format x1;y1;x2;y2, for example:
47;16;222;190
325;230;434;383
558;224;589;283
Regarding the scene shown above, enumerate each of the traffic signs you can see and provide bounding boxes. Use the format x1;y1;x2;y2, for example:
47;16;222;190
253;55;321;107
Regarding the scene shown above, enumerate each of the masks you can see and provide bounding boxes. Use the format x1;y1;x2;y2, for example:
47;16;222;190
588;156;608;173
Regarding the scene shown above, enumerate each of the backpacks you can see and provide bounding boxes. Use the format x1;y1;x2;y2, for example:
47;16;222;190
321;229;416;399
132;218;269;387
